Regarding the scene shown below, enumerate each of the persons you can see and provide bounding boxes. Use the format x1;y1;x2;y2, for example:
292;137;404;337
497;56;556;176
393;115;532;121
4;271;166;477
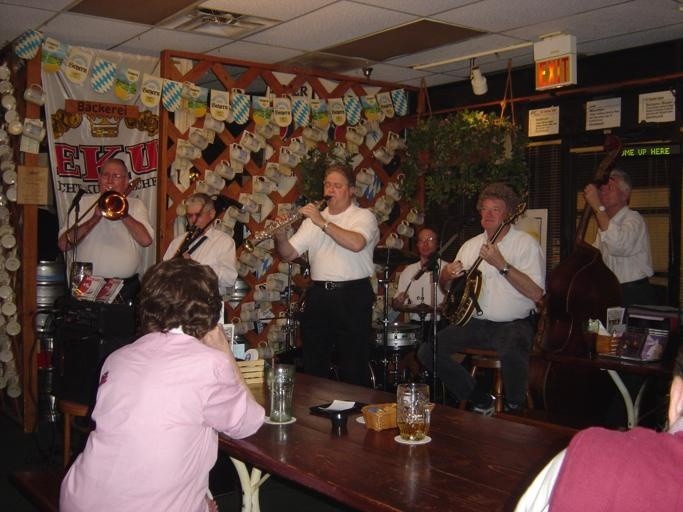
513;339;681;510
272;161;381;391
583;168;656;307
160;192;241;323
58;258;266;512
415;182;548;418
389;228;453;345
55;158;152;370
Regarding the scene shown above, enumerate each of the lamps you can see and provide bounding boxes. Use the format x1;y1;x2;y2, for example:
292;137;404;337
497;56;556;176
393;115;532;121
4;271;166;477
469;59;487;96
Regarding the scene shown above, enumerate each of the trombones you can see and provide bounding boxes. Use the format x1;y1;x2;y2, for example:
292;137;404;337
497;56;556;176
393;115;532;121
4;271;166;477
63;178;142;248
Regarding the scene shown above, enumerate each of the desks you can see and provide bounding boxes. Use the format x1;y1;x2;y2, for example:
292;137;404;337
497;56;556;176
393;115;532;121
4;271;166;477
208;364;576;512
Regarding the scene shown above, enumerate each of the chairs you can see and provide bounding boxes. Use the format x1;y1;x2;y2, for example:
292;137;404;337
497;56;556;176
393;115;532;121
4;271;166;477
456;285;551;415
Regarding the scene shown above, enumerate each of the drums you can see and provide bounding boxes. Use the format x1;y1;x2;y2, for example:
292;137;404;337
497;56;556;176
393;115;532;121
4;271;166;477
368;322;422;353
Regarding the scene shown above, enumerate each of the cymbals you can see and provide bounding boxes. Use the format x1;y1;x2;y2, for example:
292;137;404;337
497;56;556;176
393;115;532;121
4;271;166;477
399;302;443;313
373;244;421;267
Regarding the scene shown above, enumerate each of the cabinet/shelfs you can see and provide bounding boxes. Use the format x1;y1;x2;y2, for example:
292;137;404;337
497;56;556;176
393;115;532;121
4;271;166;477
573;357;676;431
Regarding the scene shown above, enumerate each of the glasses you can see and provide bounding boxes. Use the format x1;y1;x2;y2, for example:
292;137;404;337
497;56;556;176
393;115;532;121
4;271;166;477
416;235;435;242
101;172;126;180
184;211;205;217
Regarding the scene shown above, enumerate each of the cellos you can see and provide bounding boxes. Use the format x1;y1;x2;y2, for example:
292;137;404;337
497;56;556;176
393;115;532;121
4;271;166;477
528;130;623;434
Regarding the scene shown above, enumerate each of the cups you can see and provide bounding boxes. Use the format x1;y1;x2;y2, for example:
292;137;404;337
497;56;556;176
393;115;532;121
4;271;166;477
230;143;250;164
289;138;308;156
0;62;10;81
4;245;21;272
23;83;47;106
263;363;295;423
278;146;298;169
355;167;374;186
6;182;17;202
0;224;16;248
22;119;46;142
203;114;225;134
0;81;13;96
396;383;429;441
301;127;321;142
374;196;394;215
253;176;272;195
396;220;414;238
240;195;258;214
6;374;21;397
5;314;21;336
188;129;208;150
373;146;394;165
205;172;225;191
4;111;20;124
213;220;233;238
0;123;6;140
241;132;261;153
214;159;235;180
0;338;12;363
1;294;16;316
176;141;197;160
385;233;404;250
195;180;218;200
330;410;348;436
1;95;16;111
0;276;14;299
406;208;424;226
345;127;363;146
175;199;186;216
0;160;17;185
256;121;274;140
368;207;383;227
385;184;403;201
5;121;23;136
0;138;13;161
387;134;403;151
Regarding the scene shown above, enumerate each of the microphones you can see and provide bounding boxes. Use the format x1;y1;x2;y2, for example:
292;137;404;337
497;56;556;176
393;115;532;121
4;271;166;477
67;187;86;210
452;214;476;222
219;193;248;214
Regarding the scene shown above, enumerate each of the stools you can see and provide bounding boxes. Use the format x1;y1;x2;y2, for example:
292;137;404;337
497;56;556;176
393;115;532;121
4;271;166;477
57;403;93;468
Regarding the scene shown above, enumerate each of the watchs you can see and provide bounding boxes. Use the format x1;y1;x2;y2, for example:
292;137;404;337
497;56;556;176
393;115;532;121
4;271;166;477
322;221;331;232
593;205;606;215
499;262;512;278
